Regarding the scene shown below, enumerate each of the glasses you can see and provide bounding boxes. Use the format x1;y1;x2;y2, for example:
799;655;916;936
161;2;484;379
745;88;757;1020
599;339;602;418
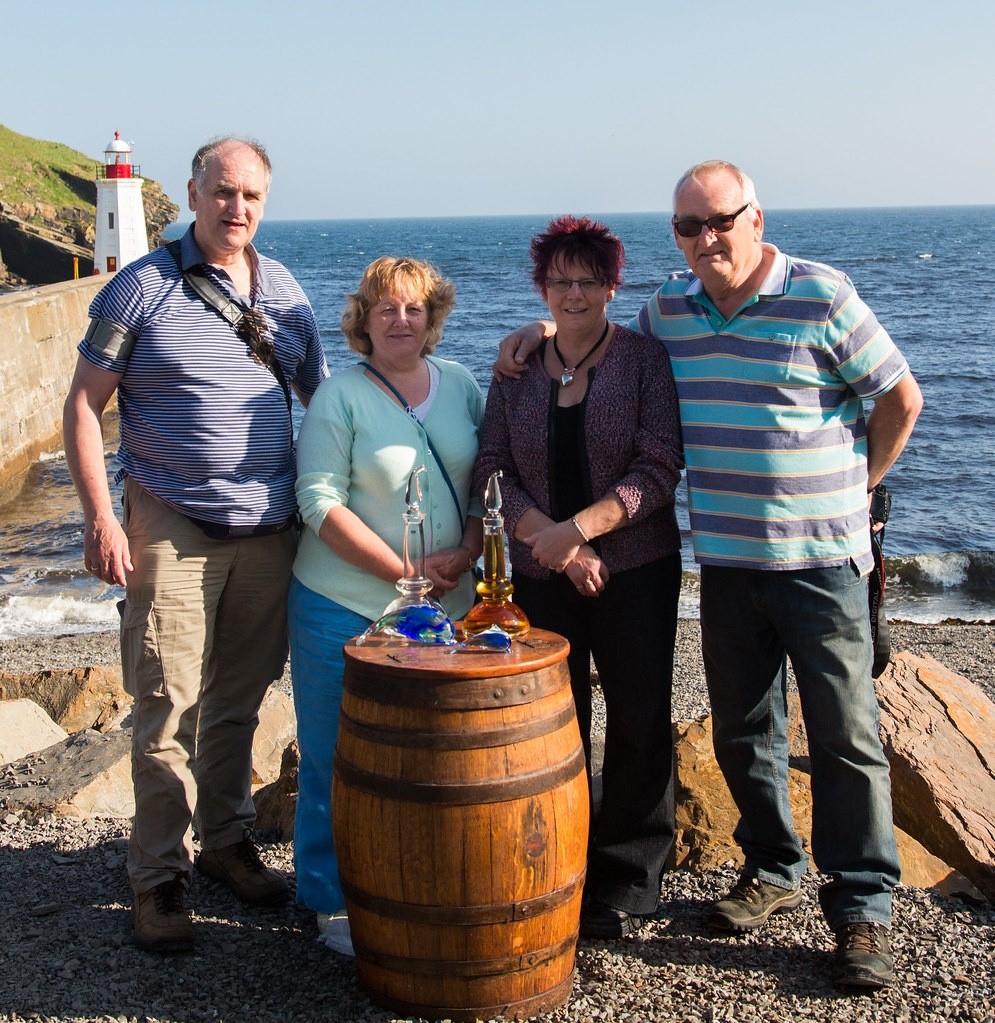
669;203;750;237
543;276;609;291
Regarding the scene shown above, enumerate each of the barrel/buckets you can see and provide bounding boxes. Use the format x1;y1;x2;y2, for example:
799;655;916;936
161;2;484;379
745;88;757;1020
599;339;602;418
331;625;591;1022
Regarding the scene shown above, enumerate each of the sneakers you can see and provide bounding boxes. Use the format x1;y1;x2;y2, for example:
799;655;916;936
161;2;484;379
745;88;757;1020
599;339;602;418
585;898;646;937
198;835;287;905
839;917;896;989
708;873;805;932
314;907;356;956
132;882;195;954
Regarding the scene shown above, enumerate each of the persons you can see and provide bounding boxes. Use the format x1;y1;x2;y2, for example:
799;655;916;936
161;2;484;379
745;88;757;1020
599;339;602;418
64;136;330;956
472;214;686;940
287;254;492;955
492;162;924;992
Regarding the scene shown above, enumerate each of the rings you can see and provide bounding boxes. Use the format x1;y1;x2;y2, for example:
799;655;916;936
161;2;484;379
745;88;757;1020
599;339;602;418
576;586;585;589
91;565;99;570
547;564;556;570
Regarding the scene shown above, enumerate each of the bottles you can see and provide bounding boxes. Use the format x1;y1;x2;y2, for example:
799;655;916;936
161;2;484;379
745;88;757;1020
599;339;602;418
382;464;445;639
462;470;531;645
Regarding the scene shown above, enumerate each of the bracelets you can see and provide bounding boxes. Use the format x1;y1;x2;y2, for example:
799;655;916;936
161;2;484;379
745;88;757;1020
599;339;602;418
571;517;589;543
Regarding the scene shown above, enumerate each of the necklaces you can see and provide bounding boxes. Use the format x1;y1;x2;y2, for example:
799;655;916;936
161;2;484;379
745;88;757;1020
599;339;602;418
553;316;609;387
